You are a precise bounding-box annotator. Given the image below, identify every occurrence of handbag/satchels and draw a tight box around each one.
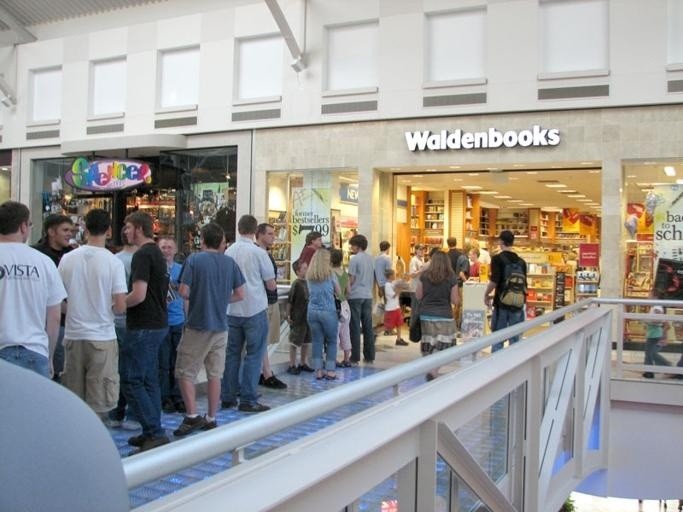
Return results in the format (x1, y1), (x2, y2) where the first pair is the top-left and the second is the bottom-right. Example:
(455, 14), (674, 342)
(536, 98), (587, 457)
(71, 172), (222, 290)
(335, 297), (341, 318)
(409, 310), (421, 342)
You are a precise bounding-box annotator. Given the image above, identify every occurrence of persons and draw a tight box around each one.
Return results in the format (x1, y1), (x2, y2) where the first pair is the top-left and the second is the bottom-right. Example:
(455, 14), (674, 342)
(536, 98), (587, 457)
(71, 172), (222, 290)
(636, 288), (683, 380)
(484, 231), (527, 353)
(638, 500), (683, 511)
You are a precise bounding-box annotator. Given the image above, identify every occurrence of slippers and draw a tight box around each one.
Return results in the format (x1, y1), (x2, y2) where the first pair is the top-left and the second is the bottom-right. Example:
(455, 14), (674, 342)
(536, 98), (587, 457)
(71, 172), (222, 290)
(326, 375), (336, 380)
(316, 374), (325, 380)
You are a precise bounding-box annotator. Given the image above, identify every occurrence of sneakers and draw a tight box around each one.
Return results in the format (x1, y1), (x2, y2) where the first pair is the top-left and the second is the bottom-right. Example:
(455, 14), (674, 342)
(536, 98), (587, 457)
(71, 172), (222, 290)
(238, 402), (270, 412)
(128, 433), (170, 456)
(164, 400), (186, 414)
(173, 414), (217, 436)
(337, 360), (352, 367)
(259, 373), (287, 389)
(221, 400), (238, 409)
(288, 365), (300, 375)
(110, 420), (141, 430)
(298, 364), (315, 372)
(396, 339), (408, 346)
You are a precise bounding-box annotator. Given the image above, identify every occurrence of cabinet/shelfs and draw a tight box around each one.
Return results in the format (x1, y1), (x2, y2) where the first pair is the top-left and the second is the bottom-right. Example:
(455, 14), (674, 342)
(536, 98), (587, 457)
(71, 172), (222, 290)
(409, 190), (601, 326)
(266, 210), (291, 283)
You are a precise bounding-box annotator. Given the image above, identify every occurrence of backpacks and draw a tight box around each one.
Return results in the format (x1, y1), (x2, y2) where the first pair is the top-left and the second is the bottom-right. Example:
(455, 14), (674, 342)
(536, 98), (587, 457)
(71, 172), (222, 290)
(496, 252), (527, 310)
